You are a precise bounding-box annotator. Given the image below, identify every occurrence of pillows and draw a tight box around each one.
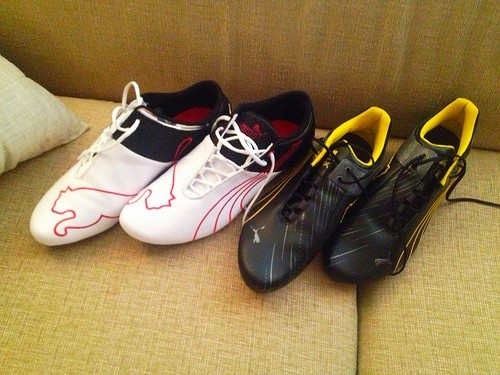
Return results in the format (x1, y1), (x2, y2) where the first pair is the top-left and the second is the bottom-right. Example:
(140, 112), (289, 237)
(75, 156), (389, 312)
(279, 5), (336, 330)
(0, 53), (91, 176)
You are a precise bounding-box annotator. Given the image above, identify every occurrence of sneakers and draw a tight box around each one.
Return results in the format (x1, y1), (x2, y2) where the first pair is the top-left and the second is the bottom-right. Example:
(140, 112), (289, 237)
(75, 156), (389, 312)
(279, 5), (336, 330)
(119, 90), (315, 245)
(322, 98), (479, 284)
(28, 80), (233, 246)
(237, 105), (393, 293)
